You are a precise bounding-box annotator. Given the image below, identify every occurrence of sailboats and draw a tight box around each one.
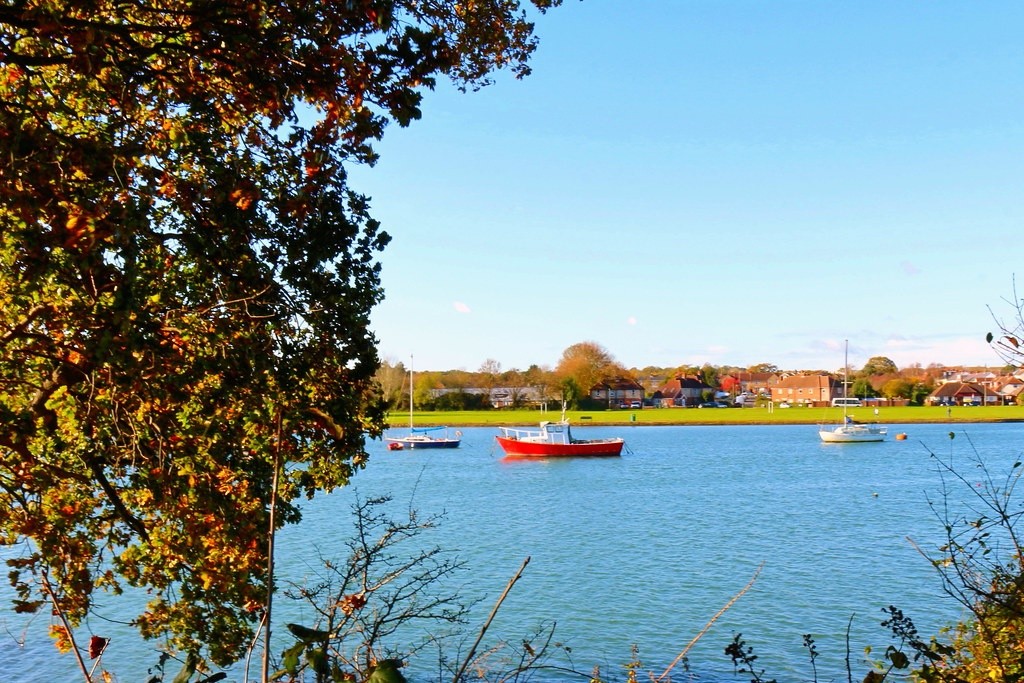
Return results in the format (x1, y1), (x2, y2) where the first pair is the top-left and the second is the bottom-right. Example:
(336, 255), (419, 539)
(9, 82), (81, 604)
(383, 353), (463, 451)
(818, 340), (889, 443)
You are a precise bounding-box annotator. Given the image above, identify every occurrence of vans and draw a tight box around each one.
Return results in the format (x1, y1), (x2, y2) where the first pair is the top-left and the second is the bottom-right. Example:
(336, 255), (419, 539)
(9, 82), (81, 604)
(831, 397), (864, 407)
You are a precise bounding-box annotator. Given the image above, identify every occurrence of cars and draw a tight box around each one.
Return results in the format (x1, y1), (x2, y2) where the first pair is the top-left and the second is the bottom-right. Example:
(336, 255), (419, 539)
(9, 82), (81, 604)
(701, 401), (728, 408)
(620, 401), (630, 409)
(742, 392), (772, 400)
(780, 402), (793, 409)
(631, 400), (641, 408)
(939, 400), (958, 406)
(963, 400), (981, 407)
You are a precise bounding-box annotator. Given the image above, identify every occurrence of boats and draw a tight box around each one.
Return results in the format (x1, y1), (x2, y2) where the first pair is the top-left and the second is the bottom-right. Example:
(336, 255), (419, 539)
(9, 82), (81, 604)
(495, 398), (625, 458)
